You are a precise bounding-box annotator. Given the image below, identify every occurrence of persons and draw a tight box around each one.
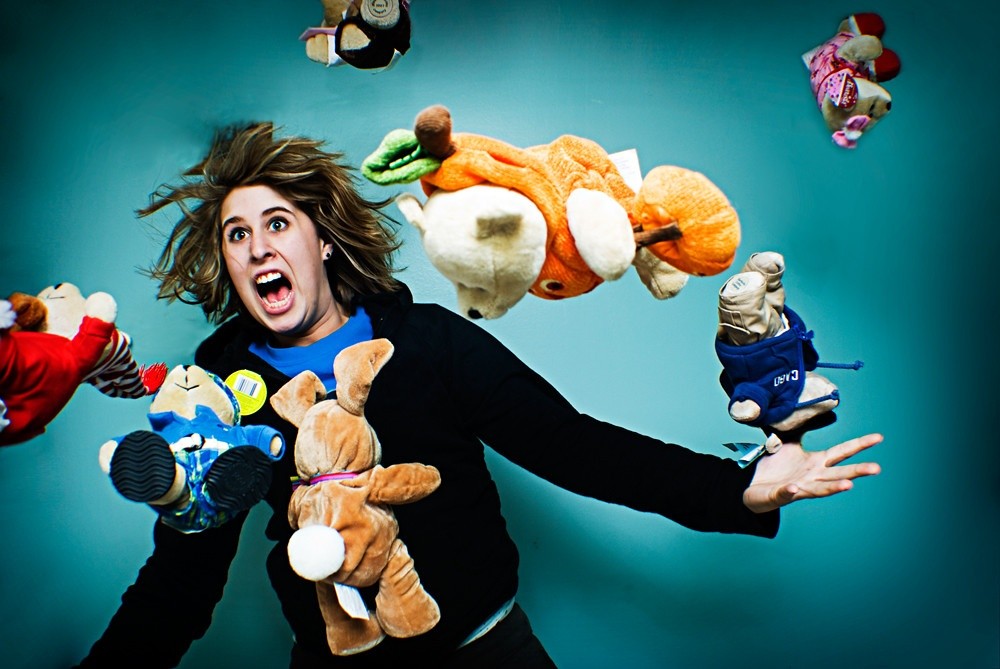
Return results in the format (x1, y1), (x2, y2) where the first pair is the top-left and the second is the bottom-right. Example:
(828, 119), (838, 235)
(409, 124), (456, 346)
(72, 120), (884, 669)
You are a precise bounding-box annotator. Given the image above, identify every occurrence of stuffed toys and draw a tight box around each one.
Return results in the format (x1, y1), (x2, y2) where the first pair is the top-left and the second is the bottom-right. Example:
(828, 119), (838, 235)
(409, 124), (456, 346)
(713, 250), (865, 454)
(359, 104), (742, 321)
(298, 0), (411, 76)
(0, 282), (169, 448)
(269, 338), (441, 655)
(800, 13), (901, 150)
(98, 365), (286, 534)
